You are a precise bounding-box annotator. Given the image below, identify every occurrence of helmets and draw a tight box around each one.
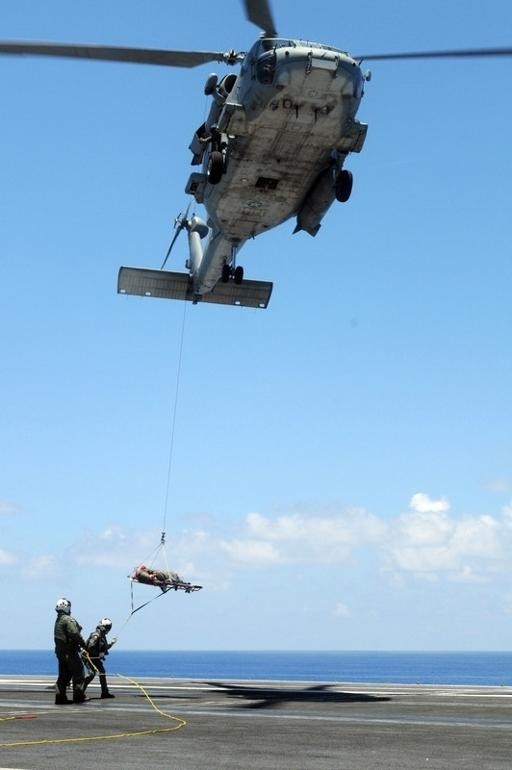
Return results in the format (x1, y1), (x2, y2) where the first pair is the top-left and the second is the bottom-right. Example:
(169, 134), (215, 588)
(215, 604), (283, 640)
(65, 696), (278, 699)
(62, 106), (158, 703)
(55, 598), (71, 615)
(97, 618), (112, 635)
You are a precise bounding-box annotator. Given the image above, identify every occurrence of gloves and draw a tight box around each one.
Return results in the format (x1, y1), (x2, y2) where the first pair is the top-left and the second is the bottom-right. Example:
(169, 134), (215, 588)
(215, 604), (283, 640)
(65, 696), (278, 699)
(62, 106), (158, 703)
(111, 634), (119, 643)
(99, 652), (104, 662)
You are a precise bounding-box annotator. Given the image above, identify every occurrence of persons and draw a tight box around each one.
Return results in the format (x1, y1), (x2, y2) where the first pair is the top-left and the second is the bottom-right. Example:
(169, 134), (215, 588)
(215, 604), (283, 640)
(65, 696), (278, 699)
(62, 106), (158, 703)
(54, 598), (117, 705)
(199, 123), (228, 153)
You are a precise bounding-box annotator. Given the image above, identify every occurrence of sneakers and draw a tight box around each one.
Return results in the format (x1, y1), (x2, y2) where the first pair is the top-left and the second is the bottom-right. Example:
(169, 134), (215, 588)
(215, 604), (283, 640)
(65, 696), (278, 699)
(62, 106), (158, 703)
(101, 693), (115, 699)
(55, 694), (90, 704)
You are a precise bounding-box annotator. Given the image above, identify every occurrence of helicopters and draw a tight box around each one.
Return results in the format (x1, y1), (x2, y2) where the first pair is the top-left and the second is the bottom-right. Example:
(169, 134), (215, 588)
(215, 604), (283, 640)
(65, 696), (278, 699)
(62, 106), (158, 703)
(0, 0), (512, 310)
